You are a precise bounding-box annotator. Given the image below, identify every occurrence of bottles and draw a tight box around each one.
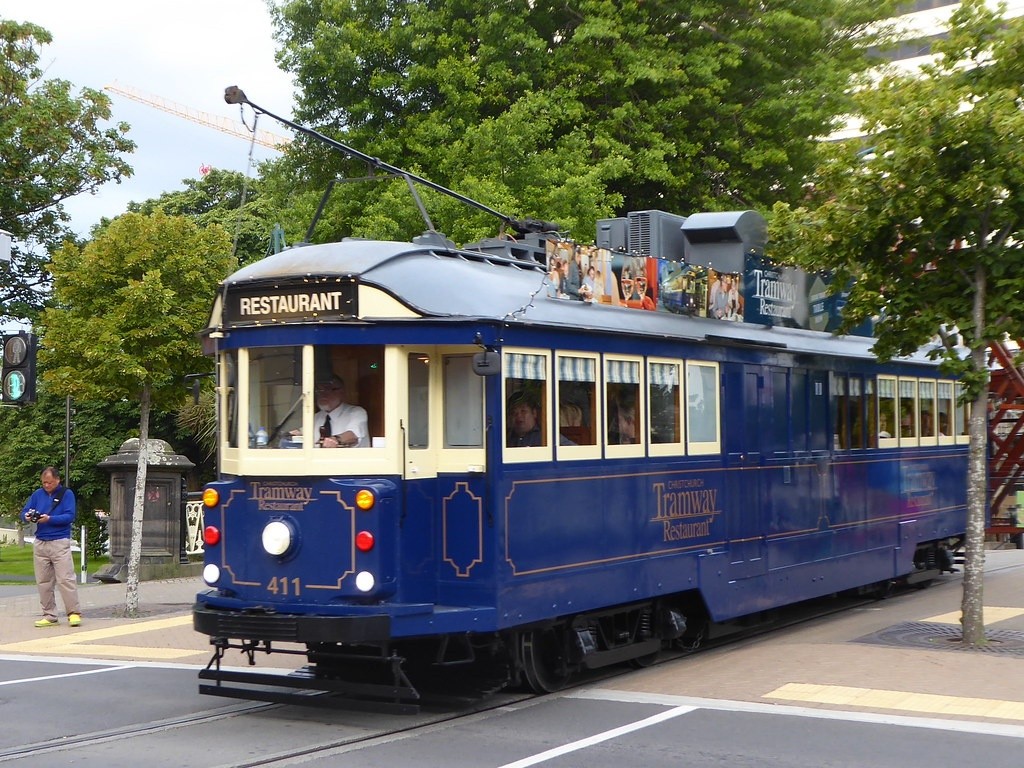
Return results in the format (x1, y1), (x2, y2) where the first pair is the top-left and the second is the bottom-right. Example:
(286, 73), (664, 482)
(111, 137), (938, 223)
(255, 426), (269, 449)
(249, 426), (254, 447)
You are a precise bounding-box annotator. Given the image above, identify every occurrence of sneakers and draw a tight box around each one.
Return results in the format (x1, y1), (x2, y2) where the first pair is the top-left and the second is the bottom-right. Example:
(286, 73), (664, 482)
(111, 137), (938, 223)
(69, 614), (81, 626)
(34, 619), (60, 626)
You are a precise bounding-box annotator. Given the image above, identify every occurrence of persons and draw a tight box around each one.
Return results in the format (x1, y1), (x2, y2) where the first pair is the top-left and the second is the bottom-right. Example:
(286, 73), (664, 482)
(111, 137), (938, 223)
(506, 376), (678, 446)
(281, 372), (373, 450)
(544, 237), (748, 323)
(21, 465), (83, 627)
(877, 397), (952, 440)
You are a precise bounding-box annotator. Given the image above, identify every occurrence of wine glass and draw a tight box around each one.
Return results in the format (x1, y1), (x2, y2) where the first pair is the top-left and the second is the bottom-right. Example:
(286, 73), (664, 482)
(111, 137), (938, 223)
(620, 258), (634, 308)
(633, 258), (649, 309)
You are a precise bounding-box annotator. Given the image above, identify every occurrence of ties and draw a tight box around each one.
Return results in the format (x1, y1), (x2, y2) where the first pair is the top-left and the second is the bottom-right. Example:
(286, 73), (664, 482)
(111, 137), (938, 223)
(324, 415), (331, 437)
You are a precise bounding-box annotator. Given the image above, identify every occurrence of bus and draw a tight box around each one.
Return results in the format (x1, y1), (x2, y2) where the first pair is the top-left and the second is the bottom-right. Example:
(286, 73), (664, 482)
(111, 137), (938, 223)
(187, 231), (969, 710)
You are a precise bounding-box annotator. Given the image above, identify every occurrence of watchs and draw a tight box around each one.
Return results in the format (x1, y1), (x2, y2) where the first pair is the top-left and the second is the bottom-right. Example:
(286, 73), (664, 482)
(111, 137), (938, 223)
(333, 435), (342, 445)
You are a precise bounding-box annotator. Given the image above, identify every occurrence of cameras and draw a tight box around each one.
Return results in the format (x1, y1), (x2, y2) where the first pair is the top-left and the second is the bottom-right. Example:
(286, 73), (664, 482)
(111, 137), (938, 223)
(28, 509), (42, 523)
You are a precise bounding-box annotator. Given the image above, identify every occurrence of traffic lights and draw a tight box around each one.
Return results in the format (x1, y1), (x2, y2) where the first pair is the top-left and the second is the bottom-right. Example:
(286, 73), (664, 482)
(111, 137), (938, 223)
(0, 330), (36, 403)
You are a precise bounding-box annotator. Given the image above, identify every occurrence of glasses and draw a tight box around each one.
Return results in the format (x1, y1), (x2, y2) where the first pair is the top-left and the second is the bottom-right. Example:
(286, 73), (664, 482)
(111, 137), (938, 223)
(315, 387), (341, 393)
